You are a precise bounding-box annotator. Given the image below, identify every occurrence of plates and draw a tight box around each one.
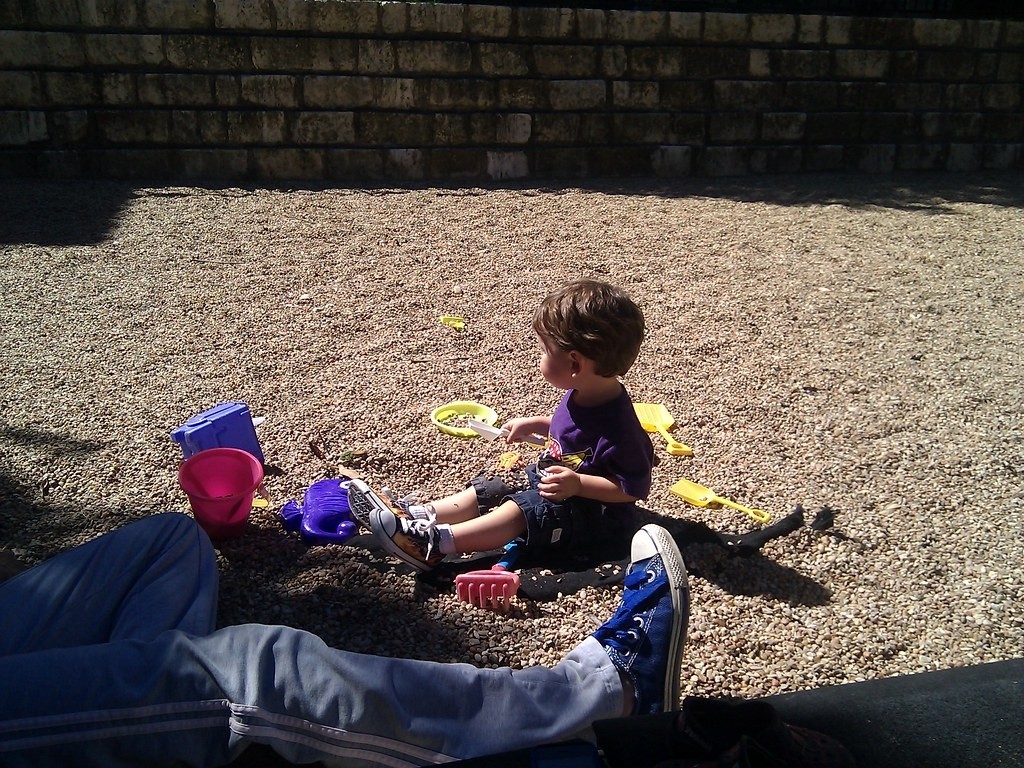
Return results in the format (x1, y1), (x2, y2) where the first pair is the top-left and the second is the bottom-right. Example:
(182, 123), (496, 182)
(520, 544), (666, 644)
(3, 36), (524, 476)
(431, 402), (498, 438)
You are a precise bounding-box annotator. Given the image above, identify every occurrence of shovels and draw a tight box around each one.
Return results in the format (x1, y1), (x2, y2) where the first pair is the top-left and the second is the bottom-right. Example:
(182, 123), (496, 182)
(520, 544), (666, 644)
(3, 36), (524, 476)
(669, 478), (771, 523)
(468, 419), (545, 446)
(632, 402), (693, 456)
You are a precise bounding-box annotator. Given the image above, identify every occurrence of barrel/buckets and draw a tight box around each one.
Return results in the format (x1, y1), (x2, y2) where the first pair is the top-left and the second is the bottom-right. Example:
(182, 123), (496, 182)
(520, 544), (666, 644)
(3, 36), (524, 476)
(178, 448), (264, 534)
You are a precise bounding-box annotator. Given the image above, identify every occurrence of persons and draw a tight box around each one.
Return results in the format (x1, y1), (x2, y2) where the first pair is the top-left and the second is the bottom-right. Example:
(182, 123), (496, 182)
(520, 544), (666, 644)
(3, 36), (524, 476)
(347, 279), (658, 574)
(0, 513), (691, 768)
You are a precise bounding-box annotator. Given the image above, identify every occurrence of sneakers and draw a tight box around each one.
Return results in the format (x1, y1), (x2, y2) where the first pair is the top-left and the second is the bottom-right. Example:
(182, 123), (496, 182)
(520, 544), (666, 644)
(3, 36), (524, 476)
(346, 479), (447, 573)
(589, 522), (691, 721)
